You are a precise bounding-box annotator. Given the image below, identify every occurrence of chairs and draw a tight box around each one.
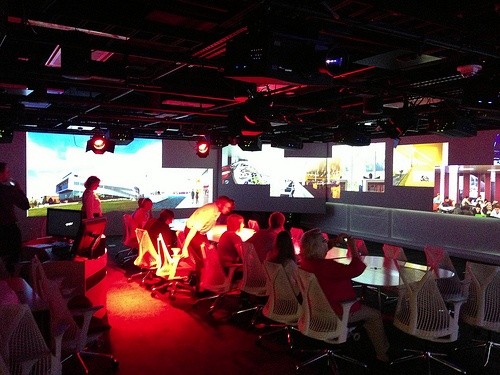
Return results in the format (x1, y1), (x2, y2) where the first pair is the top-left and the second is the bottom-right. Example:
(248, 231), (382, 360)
(388, 264), (467, 375)
(424, 245), (472, 304)
(117, 204), (409, 319)
(255, 261), (303, 354)
(0, 260), (124, 375)
(294, 268), (371, 375)
(451, 261), (500, 369)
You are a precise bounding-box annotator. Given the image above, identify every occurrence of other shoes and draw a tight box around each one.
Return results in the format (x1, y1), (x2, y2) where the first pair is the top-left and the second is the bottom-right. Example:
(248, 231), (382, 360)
(374, 357), (397, 369)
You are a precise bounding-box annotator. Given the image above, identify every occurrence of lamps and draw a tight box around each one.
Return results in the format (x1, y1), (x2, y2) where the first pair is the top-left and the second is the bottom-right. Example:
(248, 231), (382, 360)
(90, 137), (107, 155)
(197, 142), (210, 158)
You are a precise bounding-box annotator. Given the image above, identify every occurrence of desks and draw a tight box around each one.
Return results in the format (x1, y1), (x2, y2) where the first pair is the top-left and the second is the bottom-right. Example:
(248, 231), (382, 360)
(165, 217), (432, 340)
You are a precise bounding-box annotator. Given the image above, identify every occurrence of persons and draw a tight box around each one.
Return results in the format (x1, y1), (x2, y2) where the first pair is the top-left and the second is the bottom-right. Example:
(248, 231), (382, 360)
(461, 195), (500, 219)
(442, 196), (453, 214)
(137, 198), (153, 219)
(218, 214), (251, 301)
(0, 162), (30, 274)
(266, 230), (303, 305)
(182, 196), (235, 300)
(245, 212), (285, 263)
(301, 228), (393, 366)
(142, 209), (174, 267)
(131, 198), (153, 229)
(80, 176), (102, 225)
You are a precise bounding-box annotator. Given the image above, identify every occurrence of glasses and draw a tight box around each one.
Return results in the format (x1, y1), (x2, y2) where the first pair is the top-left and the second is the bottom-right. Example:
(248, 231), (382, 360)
(322, 238), (327, 243)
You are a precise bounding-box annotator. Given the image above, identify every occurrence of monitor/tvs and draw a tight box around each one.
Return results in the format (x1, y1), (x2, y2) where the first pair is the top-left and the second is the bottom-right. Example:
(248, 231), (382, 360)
(46, 208), (82, 240)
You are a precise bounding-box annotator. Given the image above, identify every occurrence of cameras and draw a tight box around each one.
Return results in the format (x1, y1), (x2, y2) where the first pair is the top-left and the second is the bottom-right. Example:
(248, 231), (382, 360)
(343, 237), (348, 244)
(9, 180), (15, 187)
